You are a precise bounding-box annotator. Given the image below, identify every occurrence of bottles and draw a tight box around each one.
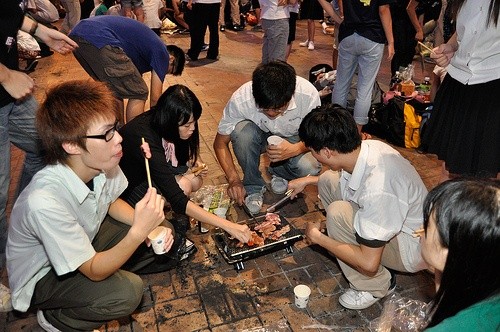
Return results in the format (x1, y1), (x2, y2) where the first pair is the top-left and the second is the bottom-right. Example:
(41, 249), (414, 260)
(420, 77), (432, 93)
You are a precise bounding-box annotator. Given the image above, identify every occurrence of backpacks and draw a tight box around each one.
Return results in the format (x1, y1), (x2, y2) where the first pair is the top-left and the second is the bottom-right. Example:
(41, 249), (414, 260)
(383, 98), (422, 149)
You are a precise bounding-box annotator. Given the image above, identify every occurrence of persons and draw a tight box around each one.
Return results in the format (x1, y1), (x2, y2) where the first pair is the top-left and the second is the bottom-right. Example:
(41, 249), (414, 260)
(213, 58), (321, 214)
(285, 103), (433, 310)
(288, 0), (343, 71)
(118, 84), (253, 244)
(6, 77), (175, 332)
(2, 0), (79, 315)
(412, 178), (500, 332)
(320, 0), (395, 140)
(390, 0), (424, 78)
(16, 0), (261, 131)
(257, 0), (291, 62)
(418, 1), (500, 185)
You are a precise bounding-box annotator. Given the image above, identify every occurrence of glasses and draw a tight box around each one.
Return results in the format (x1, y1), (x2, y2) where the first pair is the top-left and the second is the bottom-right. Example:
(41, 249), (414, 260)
(78, 119), (120, 142)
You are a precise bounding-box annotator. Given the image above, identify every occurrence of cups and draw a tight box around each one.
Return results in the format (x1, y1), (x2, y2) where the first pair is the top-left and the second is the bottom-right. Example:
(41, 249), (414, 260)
(267, 135), (284, 145)
(293, 285), (311, 308)
(148, 225), (168, 255)
(245, 193), (264, 214)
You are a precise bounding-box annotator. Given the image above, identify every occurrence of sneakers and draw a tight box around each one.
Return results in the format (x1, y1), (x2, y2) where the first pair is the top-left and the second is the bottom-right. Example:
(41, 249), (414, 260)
(308, 41), (314, 49)
(244, 185), (268, 214)
(338, 270), (397, 309)
(36, 310), (100, 332)
(271, 174), (288, 195)
(298, 40), (309, 46)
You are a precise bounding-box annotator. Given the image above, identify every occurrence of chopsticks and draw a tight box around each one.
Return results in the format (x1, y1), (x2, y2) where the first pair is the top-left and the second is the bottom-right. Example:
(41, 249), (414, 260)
(284, 173), (311, 196)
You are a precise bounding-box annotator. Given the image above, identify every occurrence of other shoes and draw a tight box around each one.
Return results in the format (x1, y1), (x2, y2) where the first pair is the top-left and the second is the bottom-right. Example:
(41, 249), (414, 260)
(233, 25), (240, 31)
(220, 25), (225, 31)
